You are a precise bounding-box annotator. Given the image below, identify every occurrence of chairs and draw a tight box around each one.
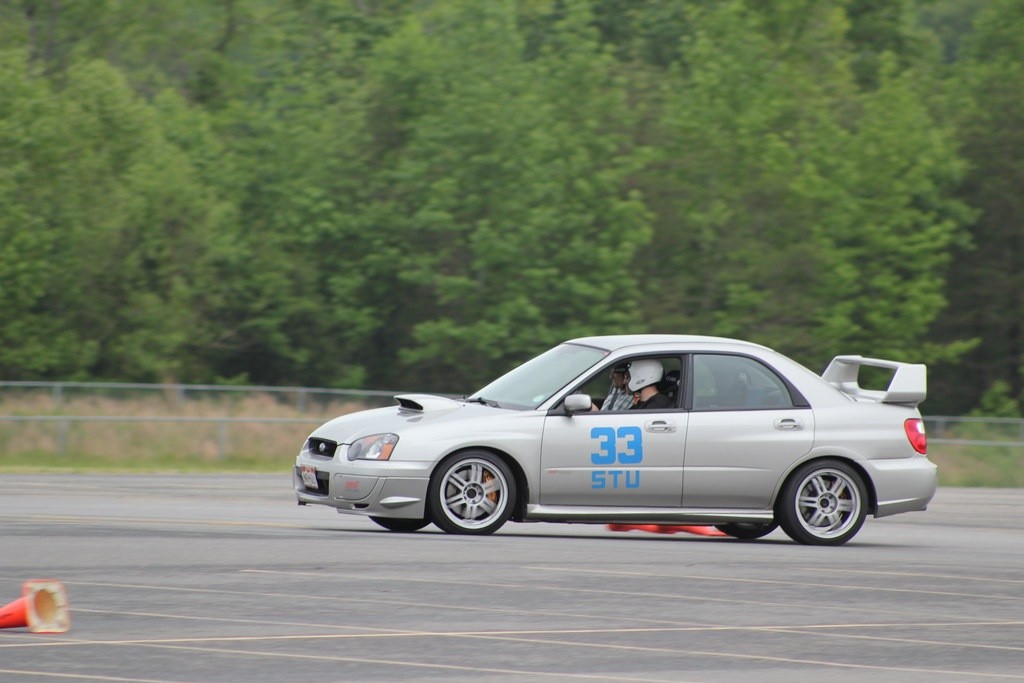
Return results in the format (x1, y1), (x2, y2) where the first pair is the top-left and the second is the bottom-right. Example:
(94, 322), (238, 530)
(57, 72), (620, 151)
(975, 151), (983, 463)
(660, 370), (680, 408)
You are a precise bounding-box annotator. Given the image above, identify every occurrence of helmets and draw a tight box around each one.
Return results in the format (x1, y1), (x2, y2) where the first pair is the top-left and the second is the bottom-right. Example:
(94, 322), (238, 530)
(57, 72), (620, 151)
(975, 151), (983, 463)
(625, 358), (663, 391)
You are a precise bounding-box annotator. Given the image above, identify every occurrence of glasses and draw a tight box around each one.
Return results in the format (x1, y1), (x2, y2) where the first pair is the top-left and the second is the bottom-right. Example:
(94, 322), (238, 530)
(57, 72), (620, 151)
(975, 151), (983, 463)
(611, 367), (626, 372)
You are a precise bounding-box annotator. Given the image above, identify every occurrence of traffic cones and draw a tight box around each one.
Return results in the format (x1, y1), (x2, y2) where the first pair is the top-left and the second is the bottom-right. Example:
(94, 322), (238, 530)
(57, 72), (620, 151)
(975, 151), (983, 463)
(0, 576), (71, 635)
(607, 523), (727, 537)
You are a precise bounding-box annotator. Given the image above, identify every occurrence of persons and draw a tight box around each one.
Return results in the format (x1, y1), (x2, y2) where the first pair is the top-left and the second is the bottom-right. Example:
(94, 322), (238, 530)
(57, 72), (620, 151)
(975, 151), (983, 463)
(568, 359), (673, 411)
(600, 363), (640, 410)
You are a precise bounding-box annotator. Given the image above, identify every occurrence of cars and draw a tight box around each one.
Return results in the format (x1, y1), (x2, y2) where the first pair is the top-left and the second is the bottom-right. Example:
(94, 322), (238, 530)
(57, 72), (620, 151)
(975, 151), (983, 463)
(293, 332), (938, 550)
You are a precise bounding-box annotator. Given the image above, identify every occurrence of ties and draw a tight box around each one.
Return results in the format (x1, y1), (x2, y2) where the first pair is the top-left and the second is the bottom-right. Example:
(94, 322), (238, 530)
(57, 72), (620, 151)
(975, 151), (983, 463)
(608, 392), (619, 411)
